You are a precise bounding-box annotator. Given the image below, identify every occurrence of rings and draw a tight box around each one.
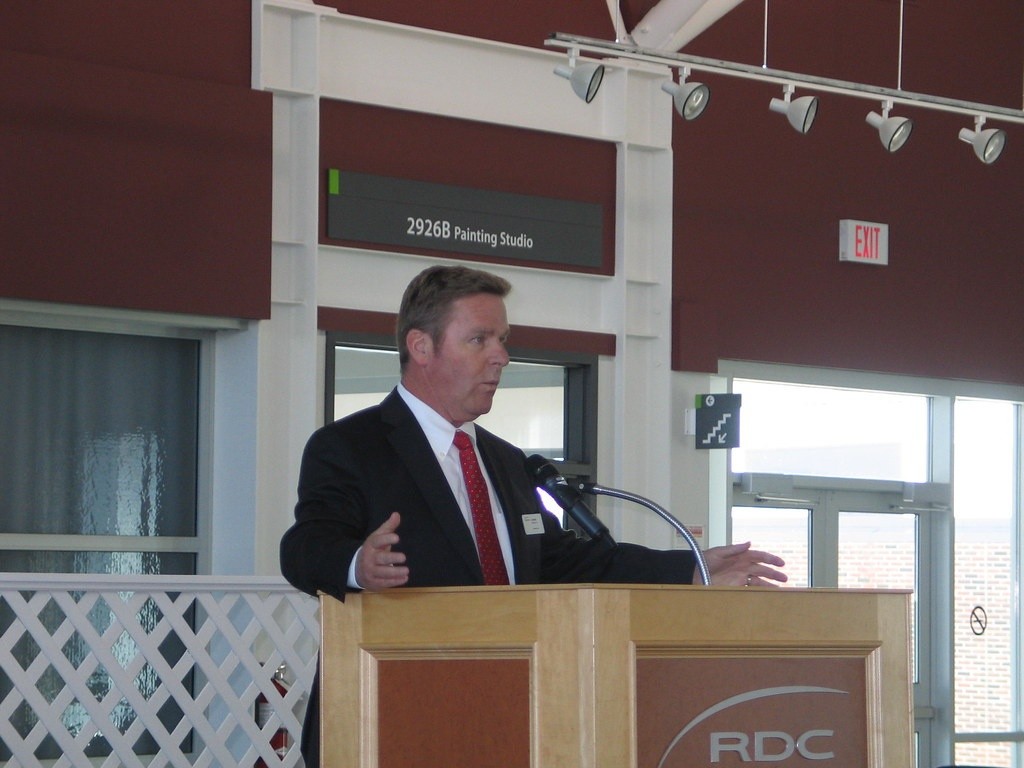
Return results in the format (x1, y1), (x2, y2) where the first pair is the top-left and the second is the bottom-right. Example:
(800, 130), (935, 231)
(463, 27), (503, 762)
(746, 575), (753, 586)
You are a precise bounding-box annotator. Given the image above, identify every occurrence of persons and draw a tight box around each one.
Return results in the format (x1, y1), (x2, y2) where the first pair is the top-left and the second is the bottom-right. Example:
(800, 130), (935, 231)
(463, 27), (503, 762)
(277, 265), (786, 768)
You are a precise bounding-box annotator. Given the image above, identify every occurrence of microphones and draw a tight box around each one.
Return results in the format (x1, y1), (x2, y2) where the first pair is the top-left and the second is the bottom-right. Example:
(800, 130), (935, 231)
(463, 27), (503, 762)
(521, 454), (618, 551)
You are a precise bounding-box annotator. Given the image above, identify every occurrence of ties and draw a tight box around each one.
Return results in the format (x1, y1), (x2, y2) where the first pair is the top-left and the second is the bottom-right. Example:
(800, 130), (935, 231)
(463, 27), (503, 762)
(452, 431), (510, 586)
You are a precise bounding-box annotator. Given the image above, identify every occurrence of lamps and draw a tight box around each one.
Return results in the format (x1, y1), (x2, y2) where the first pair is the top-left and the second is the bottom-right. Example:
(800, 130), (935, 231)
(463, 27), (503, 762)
(768, 94), (820, 135)
(551, 62), (605, 103)
(863, 110), (914, 154)
(661, 79), (710, 122)
